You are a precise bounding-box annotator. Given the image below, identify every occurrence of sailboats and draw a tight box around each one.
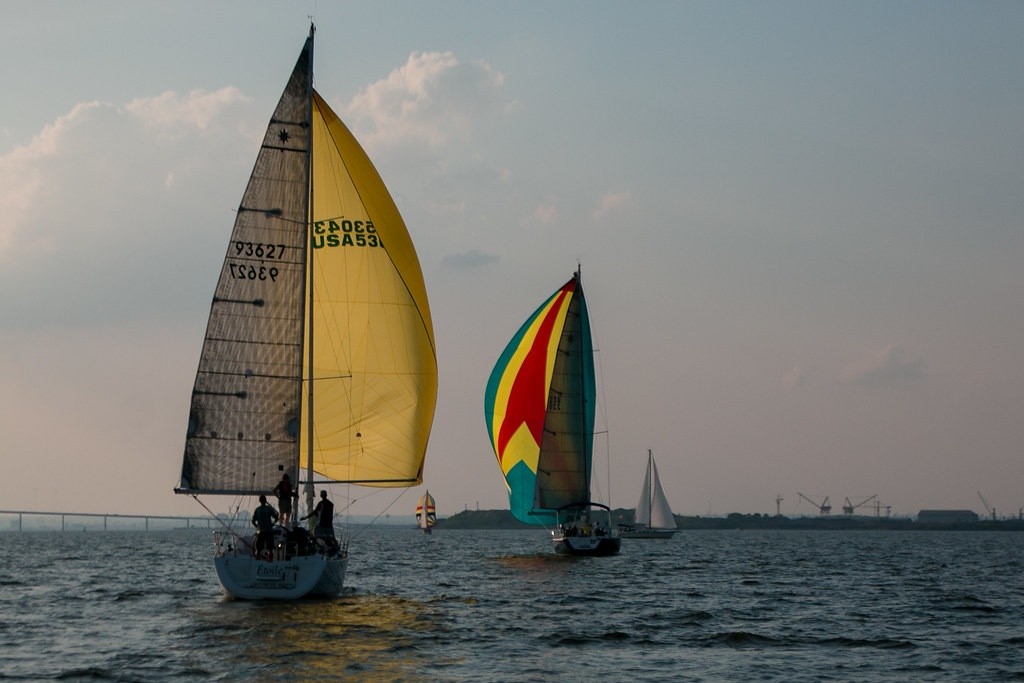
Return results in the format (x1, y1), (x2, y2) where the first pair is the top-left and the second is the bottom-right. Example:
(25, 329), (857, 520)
(484, 263), (622, 557)
(415, 489), (437, 534)
(616, 449), (679, 539)
(173, 20), (439, 602)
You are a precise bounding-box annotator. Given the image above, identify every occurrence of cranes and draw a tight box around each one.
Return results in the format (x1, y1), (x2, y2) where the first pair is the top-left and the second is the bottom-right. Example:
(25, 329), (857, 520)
(796, 491), (832, 514)
(977, 491), (995, 521)
(843, 495), (894, 517)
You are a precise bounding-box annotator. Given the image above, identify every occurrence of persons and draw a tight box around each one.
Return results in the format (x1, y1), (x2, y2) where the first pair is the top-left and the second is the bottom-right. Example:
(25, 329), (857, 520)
(300, 490), (342, 557)
(593, 522), (600, 536)
(273, 474), (299, 527)
(252, 495), (279, 561)
